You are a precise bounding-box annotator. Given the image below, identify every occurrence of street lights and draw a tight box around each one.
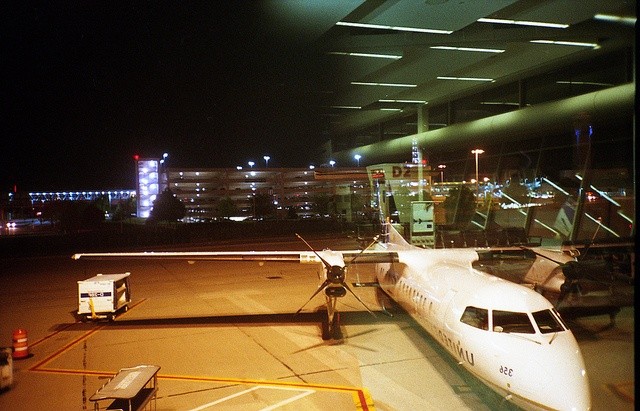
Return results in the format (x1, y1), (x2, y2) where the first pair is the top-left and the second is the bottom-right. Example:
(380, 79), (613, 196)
(247, 160), (255, 168)
(471, 148), (486, 195)
(263, 156), (271, 167)
(236, 166), (243, 170)
(329, 160), (336, 167)
(437, 165), (447, 184)
(354, 154), (362, 166)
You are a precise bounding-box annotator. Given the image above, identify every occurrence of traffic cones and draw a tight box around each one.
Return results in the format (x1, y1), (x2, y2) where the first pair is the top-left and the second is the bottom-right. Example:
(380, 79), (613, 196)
(11, 327), (32, 362)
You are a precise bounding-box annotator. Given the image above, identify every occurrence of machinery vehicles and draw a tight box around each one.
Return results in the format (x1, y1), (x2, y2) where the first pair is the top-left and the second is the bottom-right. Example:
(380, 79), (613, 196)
(76, 272), (133, 322)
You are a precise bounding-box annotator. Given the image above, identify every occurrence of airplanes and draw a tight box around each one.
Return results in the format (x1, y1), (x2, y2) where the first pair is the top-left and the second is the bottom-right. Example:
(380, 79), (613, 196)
(70, 162), (636, 411)
